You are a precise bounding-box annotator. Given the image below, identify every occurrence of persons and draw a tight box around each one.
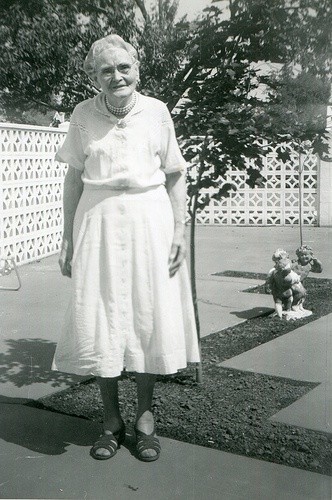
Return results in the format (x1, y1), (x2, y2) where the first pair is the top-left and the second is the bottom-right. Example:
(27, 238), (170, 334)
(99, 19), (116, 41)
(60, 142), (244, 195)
(52, 33), (202, 462)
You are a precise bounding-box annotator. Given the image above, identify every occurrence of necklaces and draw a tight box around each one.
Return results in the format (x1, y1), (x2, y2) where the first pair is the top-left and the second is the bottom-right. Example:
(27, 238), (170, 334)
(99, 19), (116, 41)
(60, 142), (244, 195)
(105, 93), (136, 116)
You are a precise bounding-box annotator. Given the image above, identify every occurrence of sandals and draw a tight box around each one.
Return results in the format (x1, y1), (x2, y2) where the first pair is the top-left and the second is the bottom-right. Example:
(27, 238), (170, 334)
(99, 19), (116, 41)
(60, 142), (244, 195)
(90, 419), (127, 461)
(134, 423), (161, 461)
